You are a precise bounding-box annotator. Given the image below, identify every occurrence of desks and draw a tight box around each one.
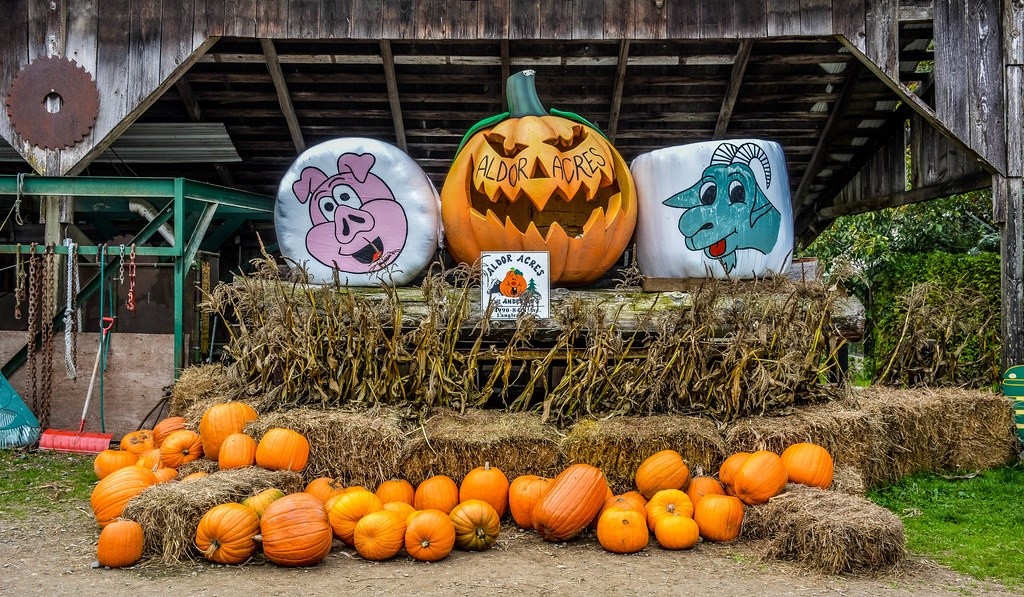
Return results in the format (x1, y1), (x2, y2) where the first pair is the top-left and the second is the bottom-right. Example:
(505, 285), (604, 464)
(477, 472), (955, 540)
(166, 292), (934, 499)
(235, 277), (865, 381)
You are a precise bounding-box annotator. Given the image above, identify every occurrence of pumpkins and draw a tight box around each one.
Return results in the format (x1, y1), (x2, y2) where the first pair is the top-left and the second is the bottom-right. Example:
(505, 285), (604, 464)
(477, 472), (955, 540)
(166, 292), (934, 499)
(90, 403), (308, 566)
(195, 442), (834, 566)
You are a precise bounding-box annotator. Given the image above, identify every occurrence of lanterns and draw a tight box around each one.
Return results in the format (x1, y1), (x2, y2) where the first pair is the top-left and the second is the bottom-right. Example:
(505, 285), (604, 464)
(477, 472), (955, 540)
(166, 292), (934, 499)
(441, 69), (637, 280)
